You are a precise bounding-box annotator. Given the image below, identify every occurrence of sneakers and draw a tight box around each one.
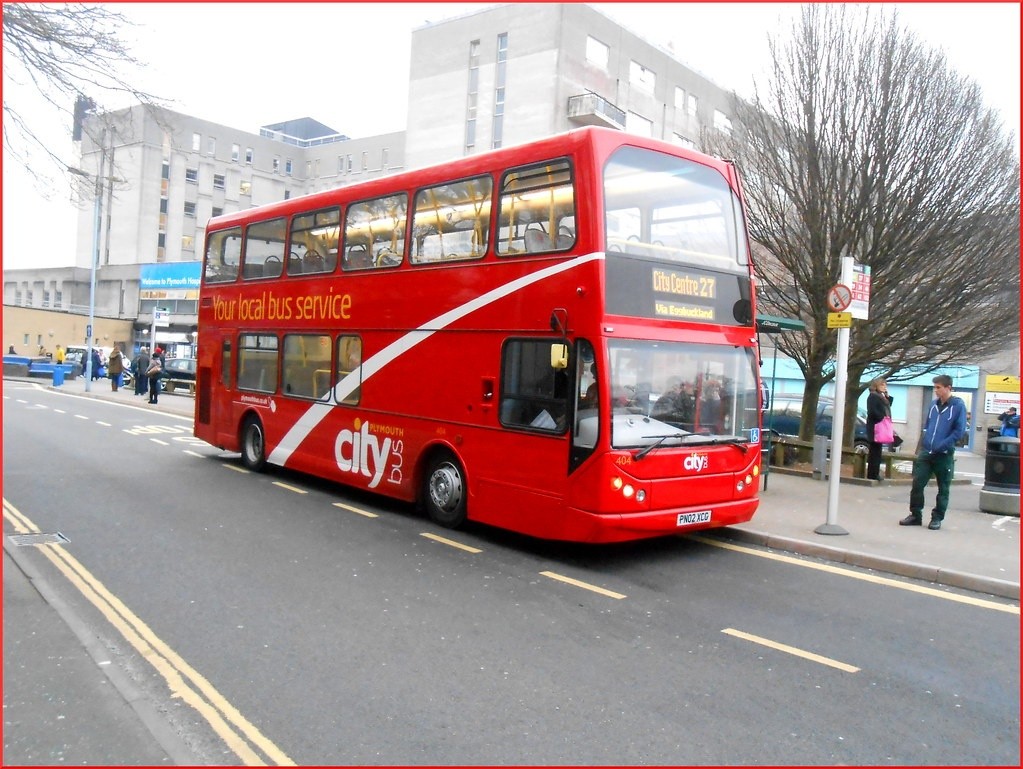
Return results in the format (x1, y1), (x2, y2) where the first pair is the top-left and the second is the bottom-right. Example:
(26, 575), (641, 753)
(929, 517), (942, 530)
(900, 515), (922, 525)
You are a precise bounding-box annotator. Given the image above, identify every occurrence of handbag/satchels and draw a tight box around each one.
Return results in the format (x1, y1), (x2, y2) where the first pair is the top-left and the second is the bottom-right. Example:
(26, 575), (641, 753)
(147, 361), (162, 377)
(98, 367), (105, 376)
(118, 374), (124, 388)
(874, 415), (895, 443)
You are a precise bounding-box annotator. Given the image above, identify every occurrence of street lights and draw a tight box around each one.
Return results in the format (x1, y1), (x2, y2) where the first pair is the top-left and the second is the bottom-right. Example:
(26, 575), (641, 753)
(67, 167), (126, 393)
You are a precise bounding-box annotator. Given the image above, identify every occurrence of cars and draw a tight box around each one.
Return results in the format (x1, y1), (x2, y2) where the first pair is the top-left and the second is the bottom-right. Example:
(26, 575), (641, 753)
(157, 357), (198, 396)
(714, 390), (905, 464)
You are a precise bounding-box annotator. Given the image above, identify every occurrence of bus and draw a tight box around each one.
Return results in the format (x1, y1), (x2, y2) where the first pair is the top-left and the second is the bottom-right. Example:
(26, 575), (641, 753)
(190, 123), (766, 559)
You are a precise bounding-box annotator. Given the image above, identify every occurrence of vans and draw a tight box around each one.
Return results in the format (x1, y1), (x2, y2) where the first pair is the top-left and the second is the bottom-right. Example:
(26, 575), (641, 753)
(64, 344), (135, 386)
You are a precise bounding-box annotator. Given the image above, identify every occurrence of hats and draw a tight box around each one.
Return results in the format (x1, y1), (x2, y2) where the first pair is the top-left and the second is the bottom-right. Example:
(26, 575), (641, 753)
(156, 348), (162, 353)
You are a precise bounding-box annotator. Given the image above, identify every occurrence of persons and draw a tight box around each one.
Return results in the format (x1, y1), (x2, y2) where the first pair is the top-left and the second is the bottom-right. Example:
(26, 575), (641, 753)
(530, 360), (624, 426)
(80, 349), (105, 381)
(900, 375), (967, 530)
(39, 345), (46, 356)
(109, 347), (123, 391)
(967, 412), (970, 428)
(650, 377), (728, 434)
(55, 345), (64, 364)
(998, 408), (1020, 437)
(867, 378), (893, 480)
(131, 346), (170, 403)
(9, 345), (16, 354)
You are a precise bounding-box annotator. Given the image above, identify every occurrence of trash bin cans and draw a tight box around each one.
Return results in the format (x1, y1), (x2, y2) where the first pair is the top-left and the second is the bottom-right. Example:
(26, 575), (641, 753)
(982, 436), (1021, 494)
(987, 426), (1001, 438)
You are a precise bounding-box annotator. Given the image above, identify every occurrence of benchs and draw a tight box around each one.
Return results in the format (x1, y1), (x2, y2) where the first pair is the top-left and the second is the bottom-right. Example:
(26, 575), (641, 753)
(225, 356), (343, 398)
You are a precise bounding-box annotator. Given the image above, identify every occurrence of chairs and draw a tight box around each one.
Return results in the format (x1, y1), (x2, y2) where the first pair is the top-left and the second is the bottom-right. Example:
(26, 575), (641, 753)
(523, 218), (665, 254)
(263, 244), (400, 276)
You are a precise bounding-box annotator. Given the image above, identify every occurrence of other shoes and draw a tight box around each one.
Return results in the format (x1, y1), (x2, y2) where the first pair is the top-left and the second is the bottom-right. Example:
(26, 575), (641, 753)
(867, 474), (884, 481)
(149, 401), (158, 404)
